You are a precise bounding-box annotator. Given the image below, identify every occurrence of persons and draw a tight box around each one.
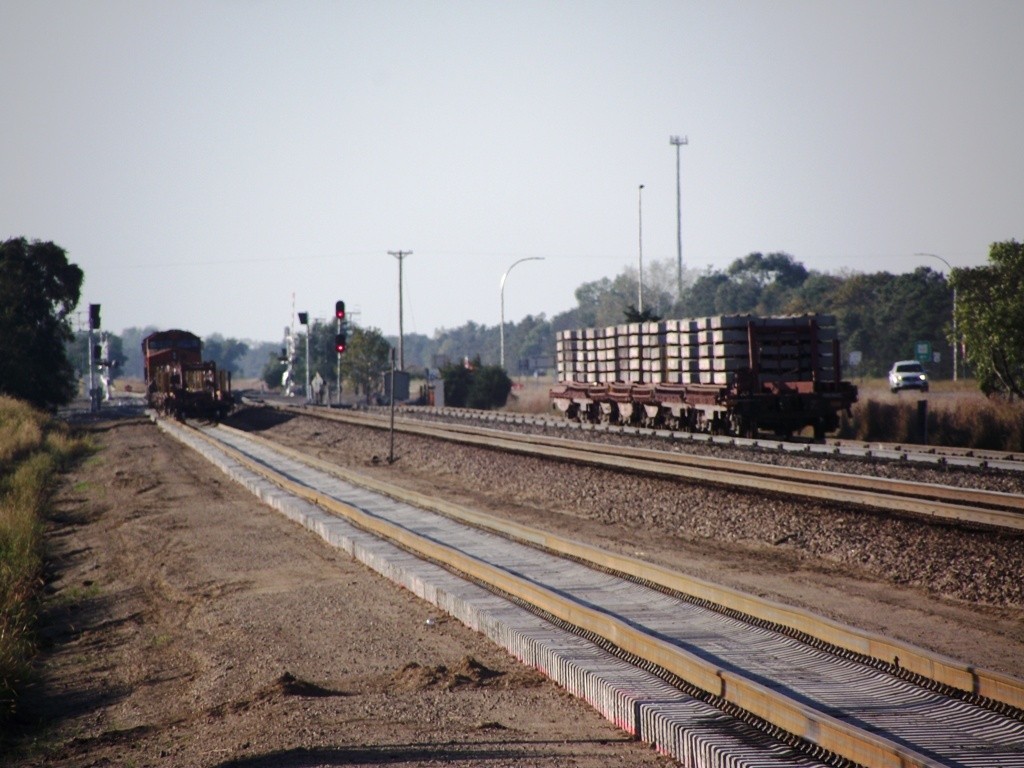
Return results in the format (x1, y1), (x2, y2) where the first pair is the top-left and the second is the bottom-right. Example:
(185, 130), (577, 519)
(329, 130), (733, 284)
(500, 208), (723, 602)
(169, 385), (188, 423)
(312, 372), (325, 404)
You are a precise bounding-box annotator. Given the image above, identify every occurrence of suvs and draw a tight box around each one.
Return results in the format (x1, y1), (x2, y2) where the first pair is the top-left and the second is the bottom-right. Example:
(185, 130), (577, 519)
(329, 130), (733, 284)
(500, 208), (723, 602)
(888, 359), (932, 395)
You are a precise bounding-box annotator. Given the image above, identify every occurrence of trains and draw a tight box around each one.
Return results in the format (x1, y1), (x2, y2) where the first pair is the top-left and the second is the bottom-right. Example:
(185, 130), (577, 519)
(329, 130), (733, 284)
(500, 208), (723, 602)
(546, 313), (859, 445)
(139, 328), (228, 427)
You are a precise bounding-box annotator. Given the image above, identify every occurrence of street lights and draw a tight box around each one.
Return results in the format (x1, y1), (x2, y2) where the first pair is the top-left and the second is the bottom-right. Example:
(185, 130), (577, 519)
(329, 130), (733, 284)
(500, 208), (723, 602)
(635, 182), (646, 319)
(914, 251), (957, 381)
(385, 249), (414, 370)
(499, 255), (548, 369)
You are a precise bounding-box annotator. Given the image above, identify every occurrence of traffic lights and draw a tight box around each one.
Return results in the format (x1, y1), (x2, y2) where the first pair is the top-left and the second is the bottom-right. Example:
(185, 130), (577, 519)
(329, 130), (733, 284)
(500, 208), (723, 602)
(91, 303), (101, 329)
(336, 300), (345, 320)
(95, 344), (102, 360)
(336, 333), (345, 353)
(297, 312), (308, 325)
